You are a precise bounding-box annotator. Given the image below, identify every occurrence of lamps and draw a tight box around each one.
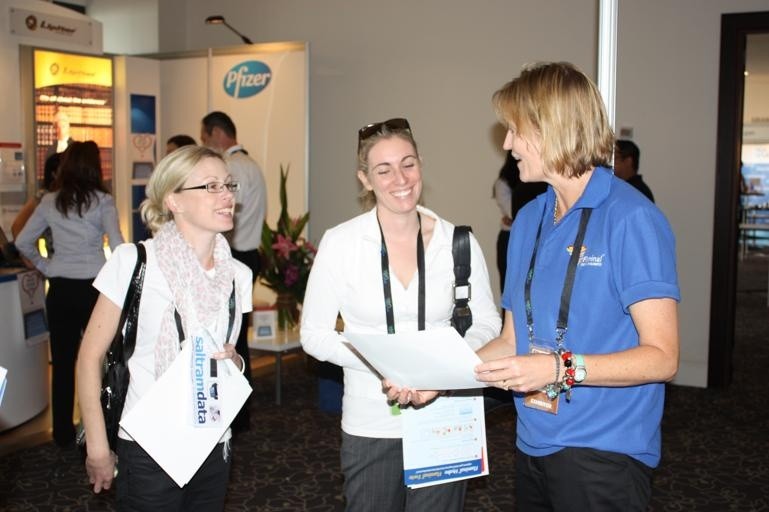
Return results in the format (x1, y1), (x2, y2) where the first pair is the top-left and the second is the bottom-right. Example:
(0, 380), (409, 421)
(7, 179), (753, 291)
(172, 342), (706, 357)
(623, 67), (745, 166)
(204, 15), (251, 46)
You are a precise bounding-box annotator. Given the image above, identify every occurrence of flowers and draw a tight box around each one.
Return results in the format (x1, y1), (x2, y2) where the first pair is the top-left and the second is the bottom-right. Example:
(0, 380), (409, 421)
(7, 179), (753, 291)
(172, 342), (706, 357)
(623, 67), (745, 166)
(256, 161), (317, 305)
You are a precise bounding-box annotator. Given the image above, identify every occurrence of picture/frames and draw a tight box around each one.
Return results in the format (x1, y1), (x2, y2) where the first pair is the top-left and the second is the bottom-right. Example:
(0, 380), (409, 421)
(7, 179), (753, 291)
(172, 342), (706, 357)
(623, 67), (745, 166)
(34, 46), (116, 198)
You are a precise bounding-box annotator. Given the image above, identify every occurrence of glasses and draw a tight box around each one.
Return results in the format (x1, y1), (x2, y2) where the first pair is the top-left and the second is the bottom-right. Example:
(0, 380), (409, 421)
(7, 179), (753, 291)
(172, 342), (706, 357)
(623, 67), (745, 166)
(358, 118), (414, 153)
(182, 181), (237, 193)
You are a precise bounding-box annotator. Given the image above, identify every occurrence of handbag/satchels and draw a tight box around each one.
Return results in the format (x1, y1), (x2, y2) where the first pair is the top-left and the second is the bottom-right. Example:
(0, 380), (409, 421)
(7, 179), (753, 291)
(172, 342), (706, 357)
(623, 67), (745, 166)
(74, 349), (128, 445)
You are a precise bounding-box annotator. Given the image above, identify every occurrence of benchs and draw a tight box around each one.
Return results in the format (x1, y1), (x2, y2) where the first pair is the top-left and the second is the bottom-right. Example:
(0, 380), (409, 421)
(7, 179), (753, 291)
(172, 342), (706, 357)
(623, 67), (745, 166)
(739, 190), (769, 260)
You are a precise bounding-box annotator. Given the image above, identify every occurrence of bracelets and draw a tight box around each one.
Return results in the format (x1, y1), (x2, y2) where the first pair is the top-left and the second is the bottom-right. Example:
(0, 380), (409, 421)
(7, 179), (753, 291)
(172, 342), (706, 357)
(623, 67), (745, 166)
(560, 351), (575, 391)
(551, 351), (560, 385)
(237, 354), (245, 373)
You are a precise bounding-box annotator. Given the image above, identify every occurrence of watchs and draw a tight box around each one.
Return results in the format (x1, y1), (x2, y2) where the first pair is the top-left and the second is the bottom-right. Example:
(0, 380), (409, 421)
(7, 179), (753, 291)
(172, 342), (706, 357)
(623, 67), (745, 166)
(573, 354), (587, 383)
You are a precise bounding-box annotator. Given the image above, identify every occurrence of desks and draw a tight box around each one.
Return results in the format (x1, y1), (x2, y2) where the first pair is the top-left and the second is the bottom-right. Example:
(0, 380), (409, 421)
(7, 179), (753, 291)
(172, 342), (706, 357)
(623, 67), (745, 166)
(248, 339), (301, 406)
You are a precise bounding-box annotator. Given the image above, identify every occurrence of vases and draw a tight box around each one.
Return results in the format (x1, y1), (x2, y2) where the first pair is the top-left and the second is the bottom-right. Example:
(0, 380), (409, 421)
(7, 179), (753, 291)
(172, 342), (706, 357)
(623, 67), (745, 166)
(274, 303), (300, 339)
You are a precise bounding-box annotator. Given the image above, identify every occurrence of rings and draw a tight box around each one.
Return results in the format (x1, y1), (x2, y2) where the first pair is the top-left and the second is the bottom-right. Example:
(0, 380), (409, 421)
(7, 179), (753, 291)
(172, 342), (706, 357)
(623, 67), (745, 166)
(502, 379), (509, 390)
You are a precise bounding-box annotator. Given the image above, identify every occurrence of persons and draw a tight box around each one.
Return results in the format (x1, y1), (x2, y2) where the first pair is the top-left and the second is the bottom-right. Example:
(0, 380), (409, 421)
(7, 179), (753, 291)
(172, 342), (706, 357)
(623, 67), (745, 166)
(615, 140), (654, 204)
(493, 150), (548, 328)
(383, 60), (681, 512)
(298, 117), (502, 512)
(74, 144), (254, 512)
(166, 134), (196, 154)
(11, 152), (65, 268)
(200, 111), (267, 434)
(13, 140), (125, 450)
